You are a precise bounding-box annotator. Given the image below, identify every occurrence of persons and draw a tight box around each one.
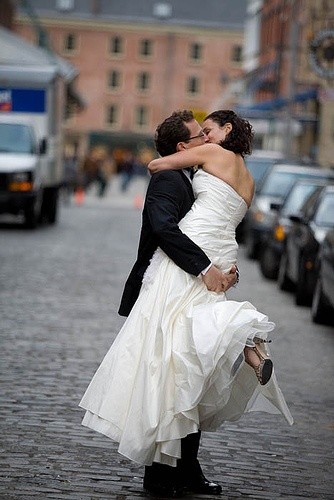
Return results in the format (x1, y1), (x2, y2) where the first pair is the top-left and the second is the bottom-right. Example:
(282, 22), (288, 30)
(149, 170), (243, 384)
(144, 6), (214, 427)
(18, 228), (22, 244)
(84, 145), (135, 197)
(77, 109), (294, 467)
(118, 110), (239, 495)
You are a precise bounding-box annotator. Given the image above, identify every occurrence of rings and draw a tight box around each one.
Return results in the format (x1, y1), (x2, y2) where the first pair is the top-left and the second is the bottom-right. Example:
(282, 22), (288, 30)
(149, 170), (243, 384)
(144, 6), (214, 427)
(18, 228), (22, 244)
(230, 269), (236, 273)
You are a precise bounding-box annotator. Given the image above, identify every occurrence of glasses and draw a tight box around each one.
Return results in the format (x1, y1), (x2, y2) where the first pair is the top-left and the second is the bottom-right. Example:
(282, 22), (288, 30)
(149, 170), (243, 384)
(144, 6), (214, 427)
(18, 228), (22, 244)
(182, 130), (204, 143)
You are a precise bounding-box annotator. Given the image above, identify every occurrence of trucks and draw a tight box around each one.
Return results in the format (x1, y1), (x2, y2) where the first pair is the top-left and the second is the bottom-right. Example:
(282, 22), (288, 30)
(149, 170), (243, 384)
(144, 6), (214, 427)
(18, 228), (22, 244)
(0, 67), (66, 227)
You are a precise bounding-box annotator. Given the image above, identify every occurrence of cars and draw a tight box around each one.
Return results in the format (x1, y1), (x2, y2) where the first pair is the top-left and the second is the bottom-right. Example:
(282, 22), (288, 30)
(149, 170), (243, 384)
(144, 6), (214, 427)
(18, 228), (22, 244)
(278, 177), (334, 326)
(235, 150), (286, 245)
(260, 179), (327, 281)
(245, 164), (334, 259)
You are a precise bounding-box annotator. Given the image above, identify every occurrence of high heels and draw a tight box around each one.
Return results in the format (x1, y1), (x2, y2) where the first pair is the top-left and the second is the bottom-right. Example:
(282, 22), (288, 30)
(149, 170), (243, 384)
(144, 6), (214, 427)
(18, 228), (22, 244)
(243, 336), (273, 386)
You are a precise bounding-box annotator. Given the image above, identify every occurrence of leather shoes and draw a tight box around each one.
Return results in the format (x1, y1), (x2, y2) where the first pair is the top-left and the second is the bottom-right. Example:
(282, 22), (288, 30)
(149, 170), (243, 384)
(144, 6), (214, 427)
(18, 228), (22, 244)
(141, 459), (223, 500)
